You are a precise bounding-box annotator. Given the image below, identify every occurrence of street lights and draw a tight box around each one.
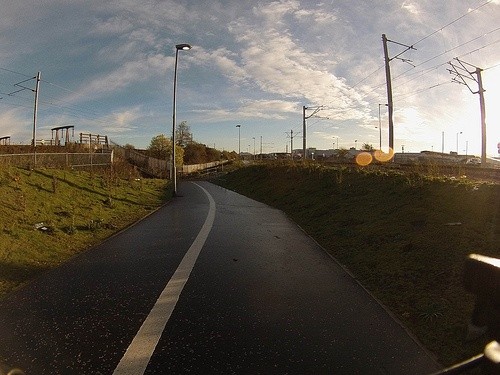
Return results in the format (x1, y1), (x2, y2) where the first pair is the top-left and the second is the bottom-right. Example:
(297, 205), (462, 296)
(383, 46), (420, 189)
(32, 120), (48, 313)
(377, 103), (389, 151)
(171, 43), (192, 196)
(236, 124), (242, 159)
